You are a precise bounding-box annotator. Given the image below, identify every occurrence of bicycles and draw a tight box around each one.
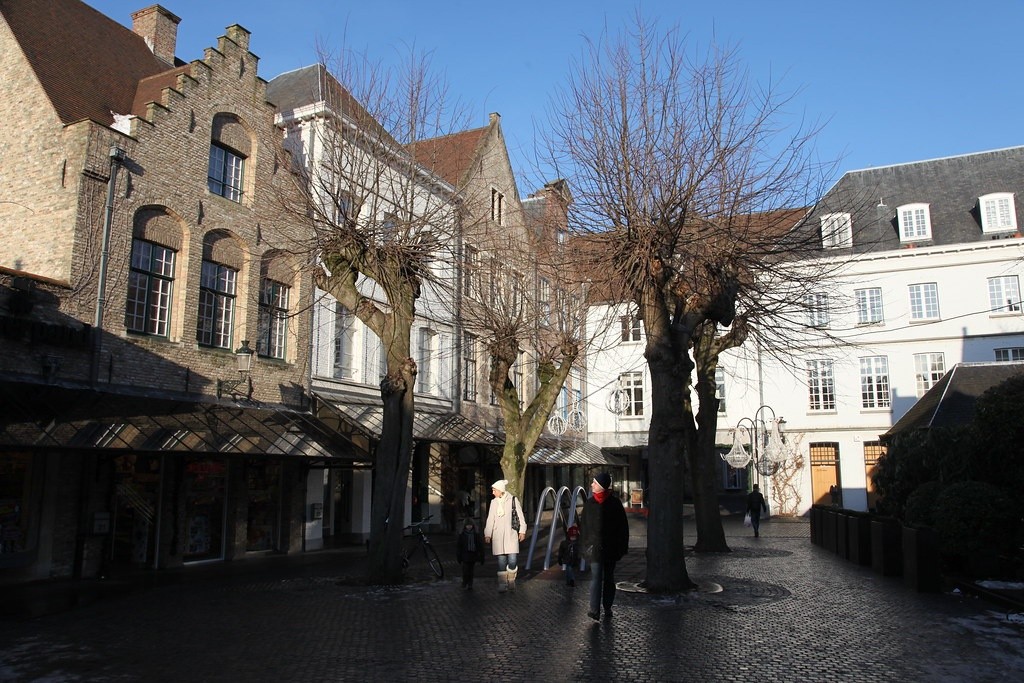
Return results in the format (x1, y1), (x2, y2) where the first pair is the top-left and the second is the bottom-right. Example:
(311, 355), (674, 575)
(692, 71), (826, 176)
(365, 514), (443, 579)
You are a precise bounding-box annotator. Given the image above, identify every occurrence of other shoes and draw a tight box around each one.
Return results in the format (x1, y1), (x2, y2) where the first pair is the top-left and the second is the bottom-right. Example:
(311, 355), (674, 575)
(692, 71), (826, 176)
(605, 608), (613, 616)
(461, 583), (467, 588)
(587, 610), (600, 620)
(468, 585), (472, 589)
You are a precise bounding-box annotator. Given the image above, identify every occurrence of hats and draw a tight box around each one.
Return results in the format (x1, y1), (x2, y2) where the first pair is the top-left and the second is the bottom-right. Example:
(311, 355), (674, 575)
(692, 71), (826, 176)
(594, 473), (611, 490)
(567, 526), (579, 540)
(464, 518), (474, 526)
(492, 480), (508, 492)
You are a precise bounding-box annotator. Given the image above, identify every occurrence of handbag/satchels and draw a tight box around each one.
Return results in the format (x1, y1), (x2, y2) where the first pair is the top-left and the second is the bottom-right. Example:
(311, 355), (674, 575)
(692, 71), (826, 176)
(743, 513), (751, 528)
(511, 496), (520, 530)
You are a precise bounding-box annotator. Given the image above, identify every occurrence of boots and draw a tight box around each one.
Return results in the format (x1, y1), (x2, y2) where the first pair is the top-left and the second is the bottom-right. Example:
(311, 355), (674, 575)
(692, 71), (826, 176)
(570, 579), (574, 587)
(566, 580), (570, 585)
(497, 571), (508, 592)
(506, 564), (518, 590)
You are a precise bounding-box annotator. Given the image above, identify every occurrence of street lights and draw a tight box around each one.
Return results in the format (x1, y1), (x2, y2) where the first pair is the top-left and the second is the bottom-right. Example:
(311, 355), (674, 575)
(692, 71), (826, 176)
(720, 405), (790, 504)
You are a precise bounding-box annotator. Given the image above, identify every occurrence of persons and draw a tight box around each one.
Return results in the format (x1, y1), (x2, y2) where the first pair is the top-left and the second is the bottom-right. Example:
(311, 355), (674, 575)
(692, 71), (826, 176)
(442, 484), (484, 521)
(830, 484), (840, 507)
(581, 473), (631, 622)
(746, 484), (766, 537)
(484, 479), (528, 594)
(556, 525), (582, 587)
(455, 518), (486, 591)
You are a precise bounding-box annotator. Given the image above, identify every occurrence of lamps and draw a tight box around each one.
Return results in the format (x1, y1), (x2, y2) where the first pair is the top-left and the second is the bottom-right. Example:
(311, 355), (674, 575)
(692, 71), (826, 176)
(724, 405), (790, 476)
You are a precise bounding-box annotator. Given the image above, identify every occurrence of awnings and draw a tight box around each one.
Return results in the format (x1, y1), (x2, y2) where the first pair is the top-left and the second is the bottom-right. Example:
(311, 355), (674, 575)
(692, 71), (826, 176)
(1, 392), (628, 480)
